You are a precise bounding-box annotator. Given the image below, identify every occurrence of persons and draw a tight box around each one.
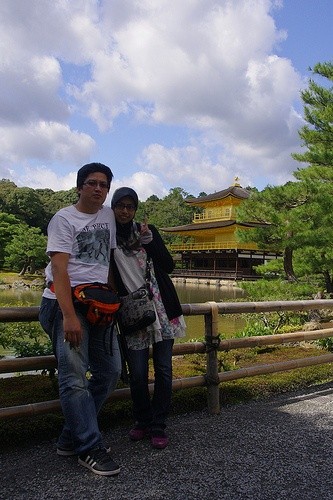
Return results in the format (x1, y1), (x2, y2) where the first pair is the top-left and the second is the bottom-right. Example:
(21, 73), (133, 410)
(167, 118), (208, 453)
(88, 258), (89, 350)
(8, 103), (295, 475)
(39, 163), (120, 476)
(106, 186), (184, 452)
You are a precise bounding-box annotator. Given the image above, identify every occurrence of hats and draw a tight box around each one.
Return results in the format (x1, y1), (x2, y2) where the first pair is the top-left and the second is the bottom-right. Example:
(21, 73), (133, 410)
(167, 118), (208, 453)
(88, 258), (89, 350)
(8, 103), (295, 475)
(111, 187), (138, 209)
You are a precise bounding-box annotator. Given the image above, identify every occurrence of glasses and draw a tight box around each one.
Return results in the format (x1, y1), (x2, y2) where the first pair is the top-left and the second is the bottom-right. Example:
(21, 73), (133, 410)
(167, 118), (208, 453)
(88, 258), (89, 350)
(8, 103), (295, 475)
(117, 204), (136, 210)
(83, 180), (109, 188)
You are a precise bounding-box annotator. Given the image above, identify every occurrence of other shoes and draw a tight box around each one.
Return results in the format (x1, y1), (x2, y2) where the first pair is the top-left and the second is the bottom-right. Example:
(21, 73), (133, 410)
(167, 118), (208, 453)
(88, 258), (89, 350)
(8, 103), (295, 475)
(151, 428), (169, 448)
(131, 426), (151, 441)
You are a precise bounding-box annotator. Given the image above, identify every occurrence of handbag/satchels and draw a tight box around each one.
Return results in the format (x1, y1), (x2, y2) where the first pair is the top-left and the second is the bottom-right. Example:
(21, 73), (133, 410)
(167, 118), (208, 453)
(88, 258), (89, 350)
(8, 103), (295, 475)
(72, 282), (156, 356)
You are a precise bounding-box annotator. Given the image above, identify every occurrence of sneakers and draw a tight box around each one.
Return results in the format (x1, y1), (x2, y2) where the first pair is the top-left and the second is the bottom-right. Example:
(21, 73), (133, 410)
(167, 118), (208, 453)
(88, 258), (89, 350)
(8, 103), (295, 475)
(57, 447), (77, 455)
(78, 446), (120, 475)
